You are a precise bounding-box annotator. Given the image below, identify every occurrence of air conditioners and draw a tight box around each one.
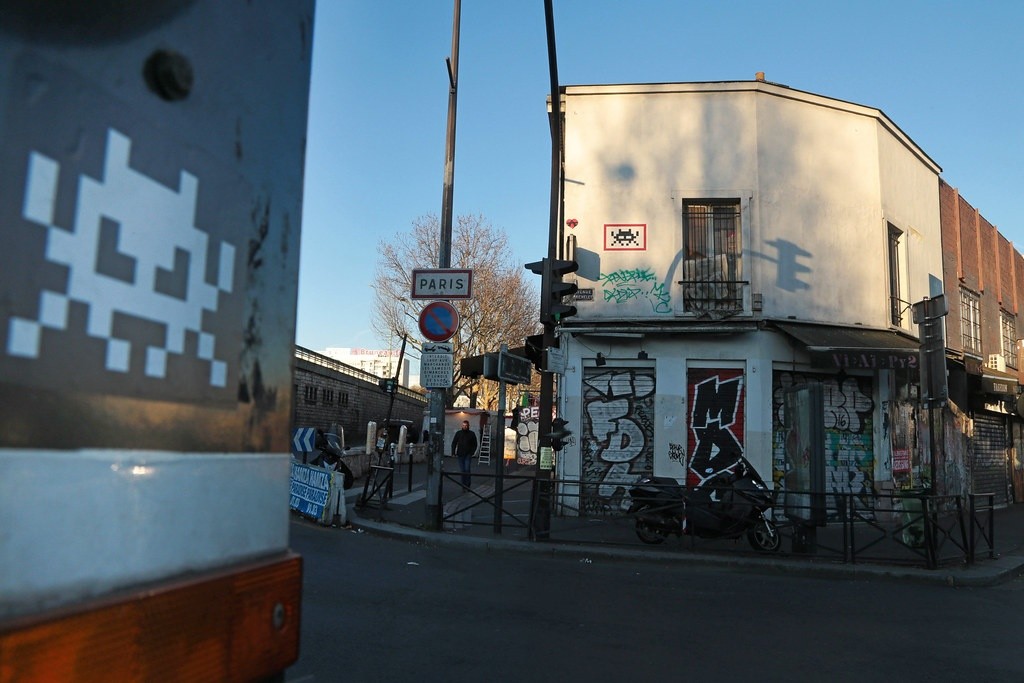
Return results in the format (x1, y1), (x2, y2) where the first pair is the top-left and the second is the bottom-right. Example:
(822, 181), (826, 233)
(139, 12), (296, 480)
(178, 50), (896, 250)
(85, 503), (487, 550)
(989, 354), (1006, 372)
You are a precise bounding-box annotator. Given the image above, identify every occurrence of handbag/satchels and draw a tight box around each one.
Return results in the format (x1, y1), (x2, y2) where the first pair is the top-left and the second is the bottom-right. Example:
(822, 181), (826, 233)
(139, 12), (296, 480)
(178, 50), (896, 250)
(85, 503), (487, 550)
(333, 457), (354, 490)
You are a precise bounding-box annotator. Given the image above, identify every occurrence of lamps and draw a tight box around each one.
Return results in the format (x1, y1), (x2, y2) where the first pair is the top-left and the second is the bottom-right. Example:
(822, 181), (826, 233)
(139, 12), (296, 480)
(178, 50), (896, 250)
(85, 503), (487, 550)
(596, 352), (606, 367)
(638, 351), (648, 359)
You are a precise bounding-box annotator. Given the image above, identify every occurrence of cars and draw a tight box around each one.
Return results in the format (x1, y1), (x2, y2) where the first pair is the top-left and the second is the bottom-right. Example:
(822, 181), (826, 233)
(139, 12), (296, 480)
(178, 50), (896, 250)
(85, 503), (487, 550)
(379, 419), (419, 444)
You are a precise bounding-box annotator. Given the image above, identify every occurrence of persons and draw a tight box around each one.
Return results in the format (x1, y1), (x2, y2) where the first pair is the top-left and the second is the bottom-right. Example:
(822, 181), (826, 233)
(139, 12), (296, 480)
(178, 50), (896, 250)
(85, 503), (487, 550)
(451, 421), (477, 492)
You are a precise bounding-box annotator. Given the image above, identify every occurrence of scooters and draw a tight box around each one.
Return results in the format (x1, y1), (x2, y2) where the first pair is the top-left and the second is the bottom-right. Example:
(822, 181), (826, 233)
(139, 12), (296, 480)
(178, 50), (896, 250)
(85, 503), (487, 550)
(306, 426), (354, 491)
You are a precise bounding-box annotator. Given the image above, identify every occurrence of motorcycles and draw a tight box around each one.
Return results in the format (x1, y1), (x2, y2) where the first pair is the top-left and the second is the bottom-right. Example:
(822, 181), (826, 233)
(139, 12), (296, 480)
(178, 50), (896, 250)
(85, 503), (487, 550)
(624, 452), (782, 553)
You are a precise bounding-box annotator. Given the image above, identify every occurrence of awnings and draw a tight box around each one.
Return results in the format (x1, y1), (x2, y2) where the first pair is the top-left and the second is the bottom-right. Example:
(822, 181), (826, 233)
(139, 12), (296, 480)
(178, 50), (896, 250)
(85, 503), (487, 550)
(461, 347), (526, 386)
(945, 354), (1018, 394)
(772, 321), (920, 353)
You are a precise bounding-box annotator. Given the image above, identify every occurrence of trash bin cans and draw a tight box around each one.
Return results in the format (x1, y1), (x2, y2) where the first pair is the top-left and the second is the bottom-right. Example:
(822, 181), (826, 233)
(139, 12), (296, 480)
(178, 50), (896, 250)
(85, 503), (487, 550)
(899, 488), (931, 548)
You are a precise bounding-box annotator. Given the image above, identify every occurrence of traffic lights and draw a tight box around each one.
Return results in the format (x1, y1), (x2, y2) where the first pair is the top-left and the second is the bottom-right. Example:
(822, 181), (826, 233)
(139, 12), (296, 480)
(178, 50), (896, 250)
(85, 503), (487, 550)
(525, 334), (544, 369)
(524, 257), (548, 324)
(552, 416), (573, 451)
(546, 255), (579, 327)
